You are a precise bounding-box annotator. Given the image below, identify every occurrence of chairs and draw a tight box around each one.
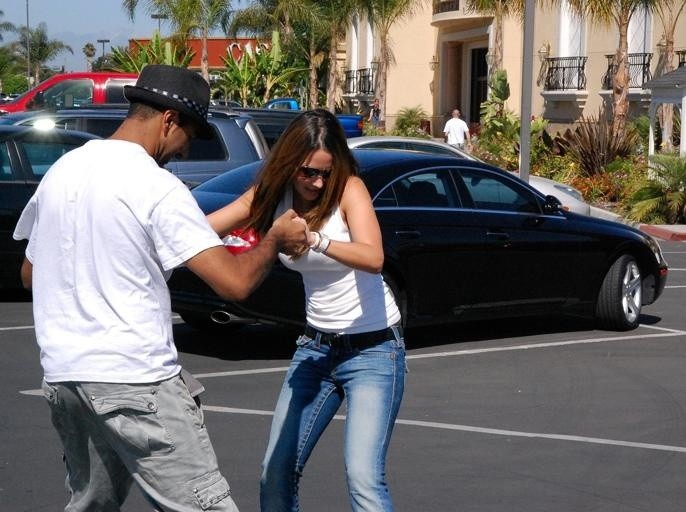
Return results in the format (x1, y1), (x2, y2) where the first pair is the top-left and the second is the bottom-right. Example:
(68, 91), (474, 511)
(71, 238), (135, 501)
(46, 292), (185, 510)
(406, 180), (438, 206)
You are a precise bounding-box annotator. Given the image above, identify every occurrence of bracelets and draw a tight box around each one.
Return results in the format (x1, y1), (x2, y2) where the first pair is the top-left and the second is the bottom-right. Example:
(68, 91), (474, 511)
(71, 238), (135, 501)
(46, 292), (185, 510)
(317, 235), (330, 253)
(309, 231), (321, 250)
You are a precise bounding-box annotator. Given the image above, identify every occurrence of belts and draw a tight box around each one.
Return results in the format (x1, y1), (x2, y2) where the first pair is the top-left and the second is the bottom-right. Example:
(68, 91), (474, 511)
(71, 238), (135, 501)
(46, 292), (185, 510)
(303, 324), (405, 347)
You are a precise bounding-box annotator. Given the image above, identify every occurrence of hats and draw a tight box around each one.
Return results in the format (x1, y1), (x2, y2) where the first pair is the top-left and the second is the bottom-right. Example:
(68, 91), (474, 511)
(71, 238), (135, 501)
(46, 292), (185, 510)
(124, 64), (214, 140)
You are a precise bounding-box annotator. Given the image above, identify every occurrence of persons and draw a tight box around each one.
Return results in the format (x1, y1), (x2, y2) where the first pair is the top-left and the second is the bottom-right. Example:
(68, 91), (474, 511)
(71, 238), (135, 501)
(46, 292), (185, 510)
(203, 109), (410, 512)
(367, 99), (381, 128)
(443, 109), (472, 152)
(11, 65), (308, 512)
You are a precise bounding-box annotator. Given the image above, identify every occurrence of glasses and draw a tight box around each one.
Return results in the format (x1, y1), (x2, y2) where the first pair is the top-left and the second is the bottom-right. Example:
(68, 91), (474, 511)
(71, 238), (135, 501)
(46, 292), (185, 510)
(296, 166), (332, 179)
